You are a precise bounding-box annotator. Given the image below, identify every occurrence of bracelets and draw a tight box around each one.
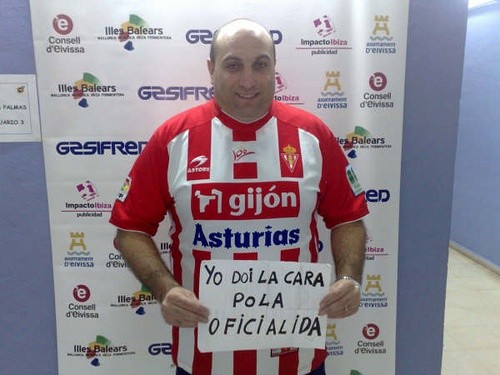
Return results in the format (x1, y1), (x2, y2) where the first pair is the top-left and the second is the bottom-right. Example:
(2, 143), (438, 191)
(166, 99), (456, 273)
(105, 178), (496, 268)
(338, 276), (364, 299)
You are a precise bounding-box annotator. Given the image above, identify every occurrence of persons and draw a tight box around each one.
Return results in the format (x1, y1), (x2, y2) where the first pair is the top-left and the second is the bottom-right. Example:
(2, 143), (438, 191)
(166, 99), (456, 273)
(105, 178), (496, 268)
(105, 19), (369, 375)
